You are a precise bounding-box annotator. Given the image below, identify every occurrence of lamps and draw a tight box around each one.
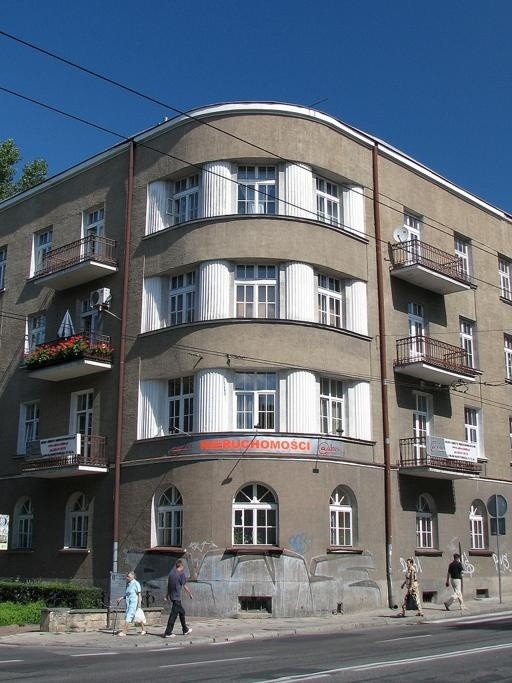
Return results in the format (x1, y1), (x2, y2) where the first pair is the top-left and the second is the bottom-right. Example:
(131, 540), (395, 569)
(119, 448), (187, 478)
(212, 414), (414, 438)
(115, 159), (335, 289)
(254, 423), (262, 432)
(325, 429), (345, 435)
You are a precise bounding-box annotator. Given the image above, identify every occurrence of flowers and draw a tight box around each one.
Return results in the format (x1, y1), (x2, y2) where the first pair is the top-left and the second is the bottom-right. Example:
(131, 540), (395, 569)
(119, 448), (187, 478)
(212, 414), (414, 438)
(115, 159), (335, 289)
(23, 336), (115, 365)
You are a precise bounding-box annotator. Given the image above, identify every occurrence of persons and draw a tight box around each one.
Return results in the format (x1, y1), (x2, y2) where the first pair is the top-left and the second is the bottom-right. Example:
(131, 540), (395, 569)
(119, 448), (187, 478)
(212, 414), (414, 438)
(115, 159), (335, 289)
(396, 558), (424, 618)
(443, 552), (468, 610)
(116, 570), (147, 637)
(164, 559), (193, 638)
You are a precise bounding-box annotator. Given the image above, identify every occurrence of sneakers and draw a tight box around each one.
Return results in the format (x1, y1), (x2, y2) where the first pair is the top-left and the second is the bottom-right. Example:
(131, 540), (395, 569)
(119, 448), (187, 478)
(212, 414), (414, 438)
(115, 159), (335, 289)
(139, 627), (150, 637)
(442, 602), (452, 612)
(414, 610), (426, 617)
(182, 628), (193, 636)
(163, 632), (176, 638)
(116, 631), (128, 639)
(397, 611), (407, 619)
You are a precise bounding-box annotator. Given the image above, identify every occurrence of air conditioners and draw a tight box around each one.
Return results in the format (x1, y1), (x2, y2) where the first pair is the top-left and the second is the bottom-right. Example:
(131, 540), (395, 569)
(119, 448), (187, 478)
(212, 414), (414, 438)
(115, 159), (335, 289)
(91, 288), (111, 309)
